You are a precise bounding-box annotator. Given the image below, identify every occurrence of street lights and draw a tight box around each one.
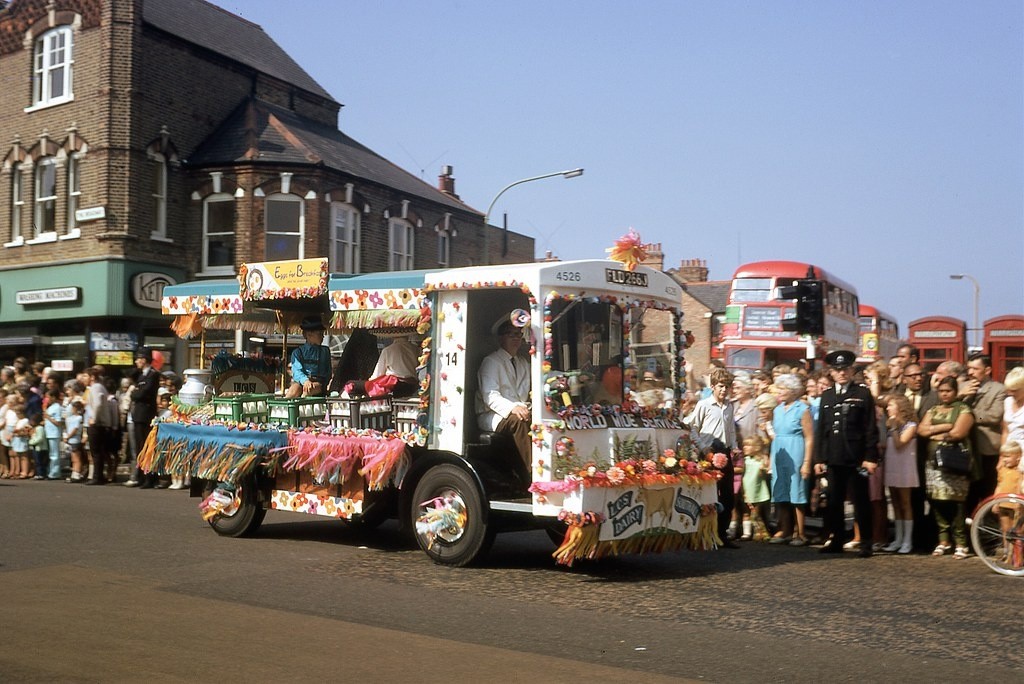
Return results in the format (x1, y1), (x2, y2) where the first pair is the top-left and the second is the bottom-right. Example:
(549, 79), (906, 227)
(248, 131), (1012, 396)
(484, 167), (586, 265)
(949, 273), (980, 347)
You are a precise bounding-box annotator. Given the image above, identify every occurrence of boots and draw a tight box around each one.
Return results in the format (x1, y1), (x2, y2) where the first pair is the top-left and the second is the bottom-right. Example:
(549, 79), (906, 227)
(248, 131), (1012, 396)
(726, 521), (738, 540)
(898, 520), (914, 554)
(741, 521), (752, 540)
(884, 520), (903, 552)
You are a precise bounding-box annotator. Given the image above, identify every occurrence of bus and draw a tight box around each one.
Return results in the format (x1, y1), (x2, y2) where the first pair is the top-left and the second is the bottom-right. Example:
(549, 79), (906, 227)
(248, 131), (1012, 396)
(723, 261), (862, 377)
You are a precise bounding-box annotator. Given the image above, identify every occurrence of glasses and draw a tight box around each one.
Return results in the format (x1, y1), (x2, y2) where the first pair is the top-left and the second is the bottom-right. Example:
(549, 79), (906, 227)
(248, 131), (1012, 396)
(501, 330), (523, 338)
(903, 372), (927, 379)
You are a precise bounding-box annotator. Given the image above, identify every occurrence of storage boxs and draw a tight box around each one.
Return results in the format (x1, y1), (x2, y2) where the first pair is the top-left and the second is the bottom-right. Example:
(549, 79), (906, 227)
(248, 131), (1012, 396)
(212, 392), (275, 424)
(266, 395), (327, 429)
(324, 395), (395, 433)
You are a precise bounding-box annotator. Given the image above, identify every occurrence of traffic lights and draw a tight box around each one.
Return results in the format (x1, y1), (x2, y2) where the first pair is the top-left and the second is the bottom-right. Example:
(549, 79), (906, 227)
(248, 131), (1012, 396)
(778, 281), (822, 335)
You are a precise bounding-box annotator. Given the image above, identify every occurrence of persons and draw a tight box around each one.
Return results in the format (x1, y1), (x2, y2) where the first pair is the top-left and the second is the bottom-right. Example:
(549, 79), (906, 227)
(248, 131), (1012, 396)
(0, 292), (1023, 569)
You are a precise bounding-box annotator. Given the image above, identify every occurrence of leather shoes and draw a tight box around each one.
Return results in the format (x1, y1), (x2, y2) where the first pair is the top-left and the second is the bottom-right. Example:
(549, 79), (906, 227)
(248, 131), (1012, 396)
(0, 465), (186, 489)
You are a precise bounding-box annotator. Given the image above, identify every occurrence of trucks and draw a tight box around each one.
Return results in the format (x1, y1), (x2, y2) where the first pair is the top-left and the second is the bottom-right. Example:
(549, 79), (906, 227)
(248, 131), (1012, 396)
(148, 257), (718, 567)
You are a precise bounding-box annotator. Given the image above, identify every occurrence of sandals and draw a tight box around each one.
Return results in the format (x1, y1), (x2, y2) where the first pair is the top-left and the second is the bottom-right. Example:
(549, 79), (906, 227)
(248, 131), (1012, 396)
(954, 547), (969, 559)
(932, 545), (952, 555)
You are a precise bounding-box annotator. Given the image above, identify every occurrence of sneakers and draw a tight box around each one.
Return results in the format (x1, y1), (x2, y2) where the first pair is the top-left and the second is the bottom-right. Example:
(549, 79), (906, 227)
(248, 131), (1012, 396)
(752, 531), (885, 557)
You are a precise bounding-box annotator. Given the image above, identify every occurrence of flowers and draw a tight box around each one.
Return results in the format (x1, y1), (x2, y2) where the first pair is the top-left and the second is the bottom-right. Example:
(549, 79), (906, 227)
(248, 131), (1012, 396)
(569, 435), (732, 486)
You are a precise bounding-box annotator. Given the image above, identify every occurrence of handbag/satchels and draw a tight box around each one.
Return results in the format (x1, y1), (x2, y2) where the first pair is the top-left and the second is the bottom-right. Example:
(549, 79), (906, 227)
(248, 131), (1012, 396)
(934, 439), (975, 476)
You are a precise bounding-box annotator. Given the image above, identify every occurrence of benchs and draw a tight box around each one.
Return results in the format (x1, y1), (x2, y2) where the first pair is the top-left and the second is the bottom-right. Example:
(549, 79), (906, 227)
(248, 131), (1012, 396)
(465, 425), (514, 452)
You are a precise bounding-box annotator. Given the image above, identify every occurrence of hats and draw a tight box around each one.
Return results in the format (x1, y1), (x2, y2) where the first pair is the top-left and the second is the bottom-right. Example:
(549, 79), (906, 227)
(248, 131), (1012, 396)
(824, 350), (857, 369)
(492, 313), (511, 337)
(133, 346), (152, 360)
(300, 316), (327, 331)
